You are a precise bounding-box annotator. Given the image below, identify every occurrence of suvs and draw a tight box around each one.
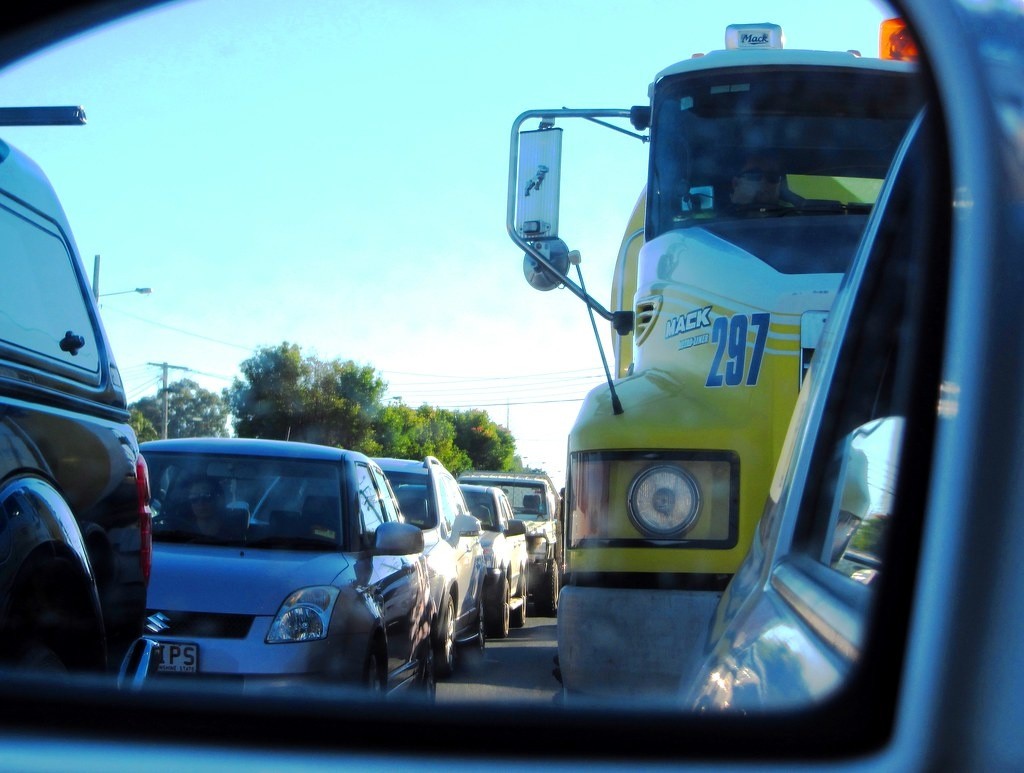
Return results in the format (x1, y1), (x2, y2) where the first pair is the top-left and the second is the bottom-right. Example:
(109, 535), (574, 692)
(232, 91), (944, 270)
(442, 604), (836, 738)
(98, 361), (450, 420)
(460, 472), (567, 616)
(290, 457), (491, 684)
(0, 145), (156, 680)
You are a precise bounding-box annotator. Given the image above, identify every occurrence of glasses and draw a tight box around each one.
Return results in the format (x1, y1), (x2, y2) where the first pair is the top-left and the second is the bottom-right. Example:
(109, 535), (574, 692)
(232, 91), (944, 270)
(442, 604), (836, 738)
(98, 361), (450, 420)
(187, 492), (215, 504)
(736, 167), (785, 185)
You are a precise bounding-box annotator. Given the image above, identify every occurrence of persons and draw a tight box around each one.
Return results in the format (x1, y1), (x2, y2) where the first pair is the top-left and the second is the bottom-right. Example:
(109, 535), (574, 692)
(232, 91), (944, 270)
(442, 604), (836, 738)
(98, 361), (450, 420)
(720, 159), (782, 211)
(162, 476), (247, 538)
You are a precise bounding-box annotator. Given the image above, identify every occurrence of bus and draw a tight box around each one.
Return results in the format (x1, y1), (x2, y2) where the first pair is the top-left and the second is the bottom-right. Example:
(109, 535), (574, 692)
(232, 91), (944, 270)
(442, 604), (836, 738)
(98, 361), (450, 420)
(503, 21), (920, 691)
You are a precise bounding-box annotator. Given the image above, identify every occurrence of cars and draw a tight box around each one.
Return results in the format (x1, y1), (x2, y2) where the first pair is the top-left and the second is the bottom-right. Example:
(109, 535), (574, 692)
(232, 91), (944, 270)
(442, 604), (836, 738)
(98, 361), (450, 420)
(391, 482), (533, 636)
(132, 437), (441, 708)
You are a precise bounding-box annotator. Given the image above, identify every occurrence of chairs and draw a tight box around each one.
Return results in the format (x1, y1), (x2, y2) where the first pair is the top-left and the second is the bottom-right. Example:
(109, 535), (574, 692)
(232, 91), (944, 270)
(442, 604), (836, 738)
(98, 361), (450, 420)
(403, 497), (428, 518)
(523, 495), (540, 510)
(301, 495), (339, 535)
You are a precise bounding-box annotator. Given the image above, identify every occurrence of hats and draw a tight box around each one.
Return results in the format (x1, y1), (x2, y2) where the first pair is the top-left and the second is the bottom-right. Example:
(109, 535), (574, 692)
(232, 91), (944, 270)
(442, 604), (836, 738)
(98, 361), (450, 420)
(732, 151), (769, 174)
